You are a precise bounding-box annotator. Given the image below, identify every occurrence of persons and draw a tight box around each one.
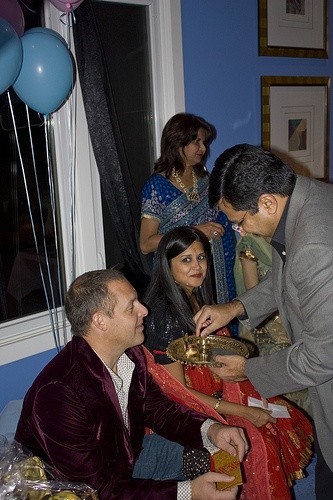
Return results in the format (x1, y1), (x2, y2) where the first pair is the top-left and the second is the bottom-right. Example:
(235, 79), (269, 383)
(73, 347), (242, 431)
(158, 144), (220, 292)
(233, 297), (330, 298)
(139, 112), (239, 339)
(193, 143), (333, 474)
(13, 268), (248, 500)
(141, 226), (313, 500)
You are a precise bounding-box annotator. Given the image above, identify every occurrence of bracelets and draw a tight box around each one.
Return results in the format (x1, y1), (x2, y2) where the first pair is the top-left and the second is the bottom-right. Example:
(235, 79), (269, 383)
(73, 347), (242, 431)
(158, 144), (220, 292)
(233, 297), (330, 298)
(214, 398), (222, 411)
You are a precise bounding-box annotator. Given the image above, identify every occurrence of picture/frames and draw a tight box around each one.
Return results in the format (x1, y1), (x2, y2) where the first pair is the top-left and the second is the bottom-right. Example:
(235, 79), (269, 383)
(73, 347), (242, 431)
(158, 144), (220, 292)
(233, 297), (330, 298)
(261, 75), (331, 183)
(258, 0), (329, 59)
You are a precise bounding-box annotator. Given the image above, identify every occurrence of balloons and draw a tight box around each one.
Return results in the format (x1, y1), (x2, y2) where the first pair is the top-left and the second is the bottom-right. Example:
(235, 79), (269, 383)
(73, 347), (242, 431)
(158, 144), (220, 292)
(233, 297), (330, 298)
(0, 0), (85, 117)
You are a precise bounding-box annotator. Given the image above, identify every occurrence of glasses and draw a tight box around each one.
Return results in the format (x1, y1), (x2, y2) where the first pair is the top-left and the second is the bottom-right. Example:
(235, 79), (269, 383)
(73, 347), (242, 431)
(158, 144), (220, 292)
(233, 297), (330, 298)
(232, 204), (250, 233)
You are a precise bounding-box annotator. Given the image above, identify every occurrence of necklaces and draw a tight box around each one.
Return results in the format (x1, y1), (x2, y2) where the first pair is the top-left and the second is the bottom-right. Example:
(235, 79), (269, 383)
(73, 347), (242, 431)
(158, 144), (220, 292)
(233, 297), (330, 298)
(172, 167), (199, 202)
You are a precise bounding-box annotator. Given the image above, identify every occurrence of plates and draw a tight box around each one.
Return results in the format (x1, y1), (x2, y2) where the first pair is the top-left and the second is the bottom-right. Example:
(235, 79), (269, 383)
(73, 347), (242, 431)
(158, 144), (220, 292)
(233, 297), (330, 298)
(166, 334), (251, 367)
